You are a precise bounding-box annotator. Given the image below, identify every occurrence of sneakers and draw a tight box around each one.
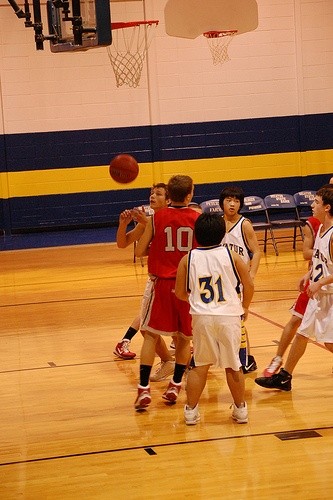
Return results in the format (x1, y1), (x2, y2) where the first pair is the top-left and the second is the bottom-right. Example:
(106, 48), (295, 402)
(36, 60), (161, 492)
(254, 369), (292, 391)
(231, 401), (249, 423)
(184, 403), (201, 425)
(134, 385), (152, 410)
(166, 342), (195, 356)
(263, 356), (282, 377)
(150, 358), (175, 382)
(113, 338), (137, 359)
(161, 381), (182, 403)
(243, 355), (257, 373)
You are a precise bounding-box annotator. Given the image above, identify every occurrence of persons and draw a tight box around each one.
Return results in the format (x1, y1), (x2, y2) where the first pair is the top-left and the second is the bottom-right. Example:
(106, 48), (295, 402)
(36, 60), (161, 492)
(262, 176), (333, 377)
(254, 187), (333, 392)
(112, 175), (262, 425)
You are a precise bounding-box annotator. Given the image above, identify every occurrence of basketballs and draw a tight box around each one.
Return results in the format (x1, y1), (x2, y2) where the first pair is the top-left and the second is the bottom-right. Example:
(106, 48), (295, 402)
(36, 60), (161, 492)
(109, 153), (139, 183)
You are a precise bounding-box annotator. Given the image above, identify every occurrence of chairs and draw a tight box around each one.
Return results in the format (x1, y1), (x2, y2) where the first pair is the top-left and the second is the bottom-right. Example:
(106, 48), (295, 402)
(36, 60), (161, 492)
(133, 190), (316, 268)
